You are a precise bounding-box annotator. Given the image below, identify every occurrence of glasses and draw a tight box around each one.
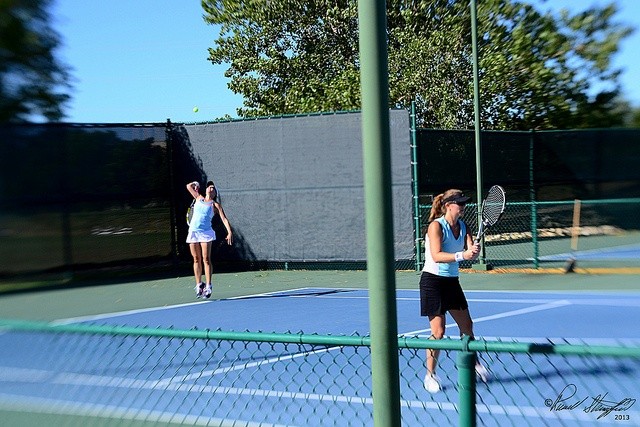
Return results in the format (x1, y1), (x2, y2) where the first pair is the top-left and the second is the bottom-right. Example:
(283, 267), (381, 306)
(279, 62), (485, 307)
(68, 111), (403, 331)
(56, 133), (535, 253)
(449, 202), (466, 206)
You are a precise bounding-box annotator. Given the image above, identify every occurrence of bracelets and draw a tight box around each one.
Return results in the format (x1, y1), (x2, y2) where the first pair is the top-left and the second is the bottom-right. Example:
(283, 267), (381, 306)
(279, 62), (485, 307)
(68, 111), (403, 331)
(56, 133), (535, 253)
(455, 251), (464, 262)
(192, 182), (199, 186)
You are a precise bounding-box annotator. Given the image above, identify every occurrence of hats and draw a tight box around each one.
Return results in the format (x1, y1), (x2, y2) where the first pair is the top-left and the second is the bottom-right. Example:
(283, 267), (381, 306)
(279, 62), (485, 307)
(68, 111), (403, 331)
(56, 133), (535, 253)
(206, 180), (217, 192)
(439, 194), (471, 203)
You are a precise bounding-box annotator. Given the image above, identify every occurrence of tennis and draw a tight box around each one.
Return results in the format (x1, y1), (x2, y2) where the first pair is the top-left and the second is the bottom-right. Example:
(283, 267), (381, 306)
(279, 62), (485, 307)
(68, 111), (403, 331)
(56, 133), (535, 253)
(193, 107), (199, 112)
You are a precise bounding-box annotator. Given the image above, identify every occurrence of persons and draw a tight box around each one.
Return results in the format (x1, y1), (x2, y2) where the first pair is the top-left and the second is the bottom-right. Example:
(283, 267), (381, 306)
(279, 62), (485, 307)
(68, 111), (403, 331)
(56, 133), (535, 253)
(419, 190), (488, 392)
(186, 181), (233, 298)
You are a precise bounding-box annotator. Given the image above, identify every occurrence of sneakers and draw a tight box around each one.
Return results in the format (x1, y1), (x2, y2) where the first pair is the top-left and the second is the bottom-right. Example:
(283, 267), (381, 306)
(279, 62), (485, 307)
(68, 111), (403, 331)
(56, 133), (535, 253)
(194, 282), (205, 298)
(203, 285), (212, 299)
(474, 358), (488, 383)
(423, 372), (442, 393)
